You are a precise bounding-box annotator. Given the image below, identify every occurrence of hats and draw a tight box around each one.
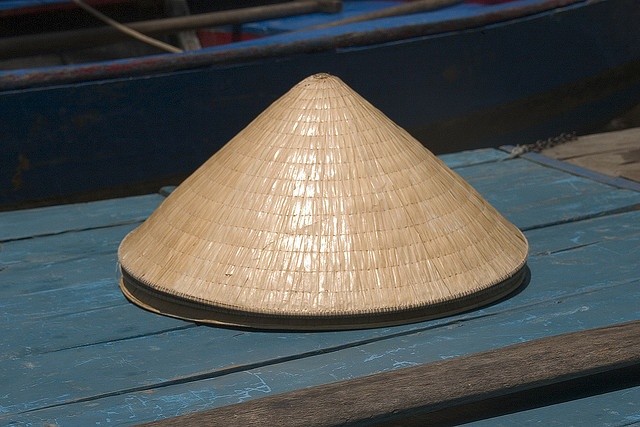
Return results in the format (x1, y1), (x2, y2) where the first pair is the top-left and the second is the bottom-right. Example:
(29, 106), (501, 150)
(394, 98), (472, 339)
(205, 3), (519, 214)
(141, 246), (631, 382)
(118, 74), (530, 332)
